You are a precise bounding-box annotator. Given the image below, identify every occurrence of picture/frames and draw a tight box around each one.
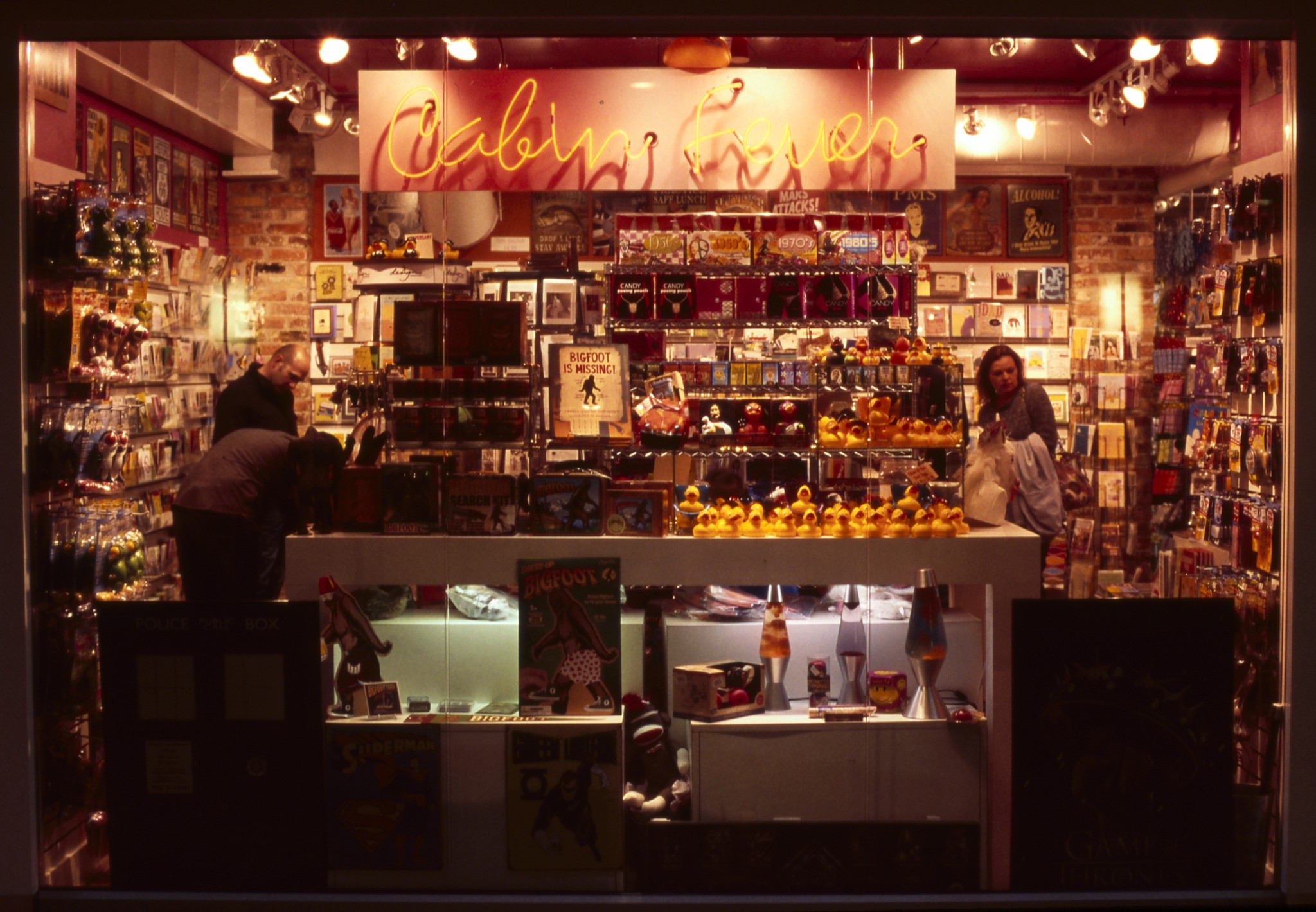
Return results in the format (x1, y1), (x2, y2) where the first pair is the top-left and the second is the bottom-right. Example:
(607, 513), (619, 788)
(940, 172), (1070, 262)
(549, 338), (634, 441)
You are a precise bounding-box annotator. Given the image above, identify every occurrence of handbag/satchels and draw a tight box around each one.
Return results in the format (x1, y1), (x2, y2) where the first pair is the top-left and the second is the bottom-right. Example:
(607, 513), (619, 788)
(1050, 445), (1096, 509)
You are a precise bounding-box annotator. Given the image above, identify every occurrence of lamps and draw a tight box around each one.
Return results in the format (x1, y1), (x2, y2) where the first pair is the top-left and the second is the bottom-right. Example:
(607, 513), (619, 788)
(949, 36), (1223, 136)
(659, 36), (733, 76)
(231, 34), (481, 140)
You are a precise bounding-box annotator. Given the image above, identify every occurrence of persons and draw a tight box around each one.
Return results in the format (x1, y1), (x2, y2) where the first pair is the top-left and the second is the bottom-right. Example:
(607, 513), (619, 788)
(978, 344), (1060, 599)
(702, 404), (732, 435)
(213, 345), (311, 599)
(173, 422), (343, 597)
